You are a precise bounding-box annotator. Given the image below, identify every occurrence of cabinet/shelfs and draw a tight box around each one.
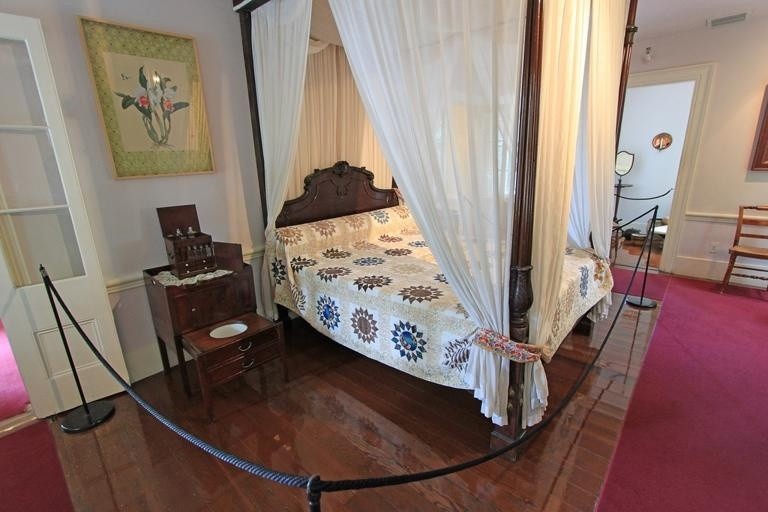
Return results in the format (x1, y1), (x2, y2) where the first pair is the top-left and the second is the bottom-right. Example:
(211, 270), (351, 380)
(142, 263), (293, 424)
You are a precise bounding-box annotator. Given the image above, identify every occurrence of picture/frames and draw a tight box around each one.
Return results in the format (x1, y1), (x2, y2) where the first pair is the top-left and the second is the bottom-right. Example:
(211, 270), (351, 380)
(79, 14), (217, 179)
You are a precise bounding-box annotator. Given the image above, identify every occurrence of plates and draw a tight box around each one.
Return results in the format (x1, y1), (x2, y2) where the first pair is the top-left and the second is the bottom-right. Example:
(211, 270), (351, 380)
(209, 322), (248, 340)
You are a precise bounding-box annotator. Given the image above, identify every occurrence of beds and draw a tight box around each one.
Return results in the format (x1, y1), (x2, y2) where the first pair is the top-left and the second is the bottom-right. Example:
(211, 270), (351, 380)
(278, 161), (596, 465)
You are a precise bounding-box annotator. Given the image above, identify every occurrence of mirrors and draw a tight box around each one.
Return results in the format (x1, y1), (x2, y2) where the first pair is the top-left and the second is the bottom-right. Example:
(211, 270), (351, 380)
(615, 151), (635, 176)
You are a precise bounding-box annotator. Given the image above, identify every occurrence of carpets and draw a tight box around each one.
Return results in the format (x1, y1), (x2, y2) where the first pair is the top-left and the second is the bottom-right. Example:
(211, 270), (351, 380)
(2, 418), (75, 512)
(595, 264), (766, 511)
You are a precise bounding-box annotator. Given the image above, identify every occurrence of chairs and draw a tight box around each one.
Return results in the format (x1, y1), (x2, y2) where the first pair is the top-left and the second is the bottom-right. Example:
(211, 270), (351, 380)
(718, 205), (767, 296)
